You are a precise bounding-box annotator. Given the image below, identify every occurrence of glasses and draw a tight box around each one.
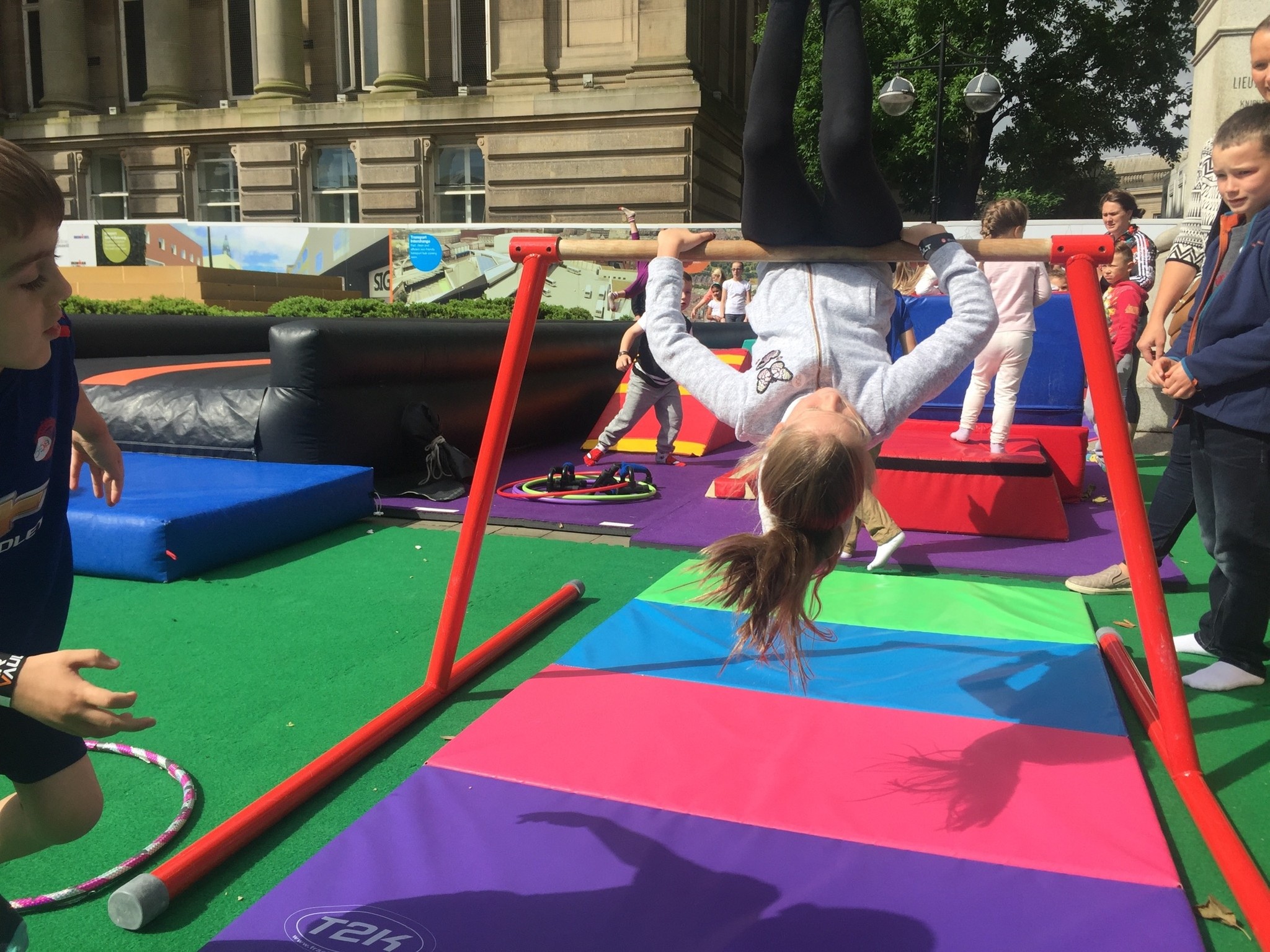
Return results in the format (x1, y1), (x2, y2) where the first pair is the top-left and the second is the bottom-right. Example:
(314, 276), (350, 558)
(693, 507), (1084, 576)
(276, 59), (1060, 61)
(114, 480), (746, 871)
(732, 268), (741, 271)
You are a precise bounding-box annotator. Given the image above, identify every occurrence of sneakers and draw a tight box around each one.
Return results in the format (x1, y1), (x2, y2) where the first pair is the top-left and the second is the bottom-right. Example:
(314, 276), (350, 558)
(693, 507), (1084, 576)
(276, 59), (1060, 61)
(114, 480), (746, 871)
(1065, 564), (1131, 595)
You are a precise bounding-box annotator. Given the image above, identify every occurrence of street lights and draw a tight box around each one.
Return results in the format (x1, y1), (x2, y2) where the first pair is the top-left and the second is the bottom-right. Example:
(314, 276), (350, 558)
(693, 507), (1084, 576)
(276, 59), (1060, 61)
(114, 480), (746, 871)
(876, 9), (1006, 225)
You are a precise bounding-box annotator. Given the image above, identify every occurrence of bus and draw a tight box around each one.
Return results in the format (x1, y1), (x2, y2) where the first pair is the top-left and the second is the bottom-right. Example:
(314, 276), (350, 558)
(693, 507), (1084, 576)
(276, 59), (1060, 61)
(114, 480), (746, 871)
(598, 285), (605, 300)
(594, 300), (603, 318)
(567, 266), (582, 275)
(545, 276), (556, 287)
(542, 287), (551, 297)
(584, 284), (591, 298)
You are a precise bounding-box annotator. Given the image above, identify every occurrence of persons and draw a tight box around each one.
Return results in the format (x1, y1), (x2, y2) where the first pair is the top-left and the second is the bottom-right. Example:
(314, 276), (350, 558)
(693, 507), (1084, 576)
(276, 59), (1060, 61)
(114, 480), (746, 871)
(0, 140), (157, 952)
(583, 0), (1269, 695)
